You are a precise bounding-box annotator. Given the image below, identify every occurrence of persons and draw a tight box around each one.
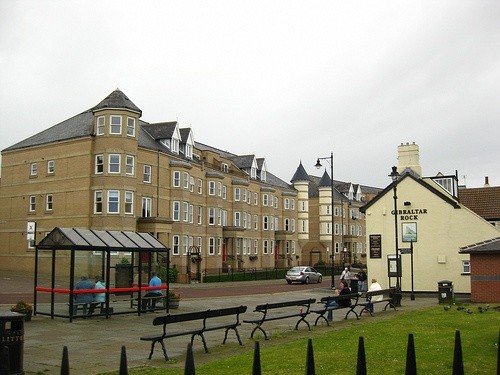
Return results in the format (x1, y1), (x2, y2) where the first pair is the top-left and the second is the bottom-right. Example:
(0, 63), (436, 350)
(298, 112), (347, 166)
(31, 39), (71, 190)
(142, 272), (161, 314)
(87, 275), (106, 317)
(365, 278), (383, 313)
(324, 280), (351, 322)
(357, 266), (367, 291)
(71, 273), (94, 318)
(340, 268), (350, 287)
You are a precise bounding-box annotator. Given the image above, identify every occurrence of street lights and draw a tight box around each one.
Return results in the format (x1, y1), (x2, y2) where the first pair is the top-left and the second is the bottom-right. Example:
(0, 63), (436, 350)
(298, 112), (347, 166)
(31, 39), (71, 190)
(388, 165), (400, 287)
(313, 152), (335, 290)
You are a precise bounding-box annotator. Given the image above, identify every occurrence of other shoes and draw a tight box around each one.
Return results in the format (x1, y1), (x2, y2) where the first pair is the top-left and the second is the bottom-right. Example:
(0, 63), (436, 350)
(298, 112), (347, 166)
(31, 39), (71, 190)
(363, 310), (367, 313)
(327, 318), (332, 321)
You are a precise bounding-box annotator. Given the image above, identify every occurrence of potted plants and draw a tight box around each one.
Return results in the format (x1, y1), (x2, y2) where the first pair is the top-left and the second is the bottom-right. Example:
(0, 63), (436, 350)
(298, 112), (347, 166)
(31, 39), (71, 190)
(162, 291), (180, 308)
(9, 301), (32, 322)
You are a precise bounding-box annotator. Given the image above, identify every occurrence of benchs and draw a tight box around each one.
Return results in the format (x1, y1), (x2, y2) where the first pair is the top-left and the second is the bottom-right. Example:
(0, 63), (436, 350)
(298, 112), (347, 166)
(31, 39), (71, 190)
(129, 296), (165, 311)
(309, 293), (359, 327)
(140, 304), (247, 361)
(359, 288), (398, 317)
(242, 298), (316, 340)
(65, 301), (105, 319)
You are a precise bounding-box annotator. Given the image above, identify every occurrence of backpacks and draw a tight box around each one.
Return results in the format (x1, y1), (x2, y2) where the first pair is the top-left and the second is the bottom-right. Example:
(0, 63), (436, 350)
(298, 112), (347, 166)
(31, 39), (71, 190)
(359, 271), (367, 281)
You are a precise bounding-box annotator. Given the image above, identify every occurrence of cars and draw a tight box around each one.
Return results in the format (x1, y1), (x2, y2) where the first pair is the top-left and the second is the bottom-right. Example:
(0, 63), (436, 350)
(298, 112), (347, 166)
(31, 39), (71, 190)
(285, 265), (322, 285)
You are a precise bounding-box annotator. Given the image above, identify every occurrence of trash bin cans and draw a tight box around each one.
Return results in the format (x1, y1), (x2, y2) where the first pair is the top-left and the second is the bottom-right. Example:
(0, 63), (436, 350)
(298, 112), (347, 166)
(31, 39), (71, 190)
(0, 311), (26, 375)
(438, 280), (454, 304)
